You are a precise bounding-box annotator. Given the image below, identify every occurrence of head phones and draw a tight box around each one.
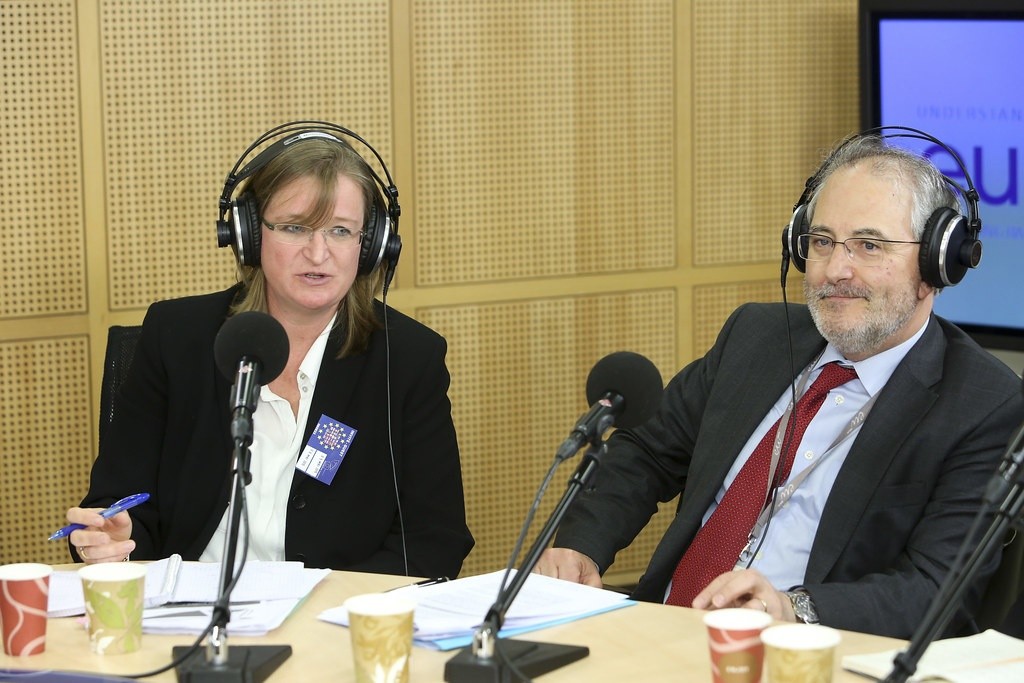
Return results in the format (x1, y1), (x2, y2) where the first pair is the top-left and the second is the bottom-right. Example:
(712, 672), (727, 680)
(788, 127), (982, 289)
(216, 120), (403, 277)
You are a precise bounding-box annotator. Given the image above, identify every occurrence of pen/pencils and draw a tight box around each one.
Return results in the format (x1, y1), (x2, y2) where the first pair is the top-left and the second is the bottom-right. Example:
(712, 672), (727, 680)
(383, 576), (449, 594)
(46, 492), (150, 541)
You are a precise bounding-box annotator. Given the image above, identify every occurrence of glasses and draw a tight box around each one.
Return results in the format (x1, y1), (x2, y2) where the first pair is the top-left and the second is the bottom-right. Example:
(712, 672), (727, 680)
(259, 216), (368, 248)
(797, 234), (921, 268)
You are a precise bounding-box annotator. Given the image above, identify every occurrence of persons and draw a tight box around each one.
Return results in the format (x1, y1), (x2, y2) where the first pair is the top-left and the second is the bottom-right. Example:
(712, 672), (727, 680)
(67, 120), (476, 581)
(533, 124), (1024, 641)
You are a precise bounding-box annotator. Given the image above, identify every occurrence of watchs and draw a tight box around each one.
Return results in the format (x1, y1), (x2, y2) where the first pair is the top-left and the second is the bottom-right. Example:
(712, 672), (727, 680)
(784, 590), (820, 625)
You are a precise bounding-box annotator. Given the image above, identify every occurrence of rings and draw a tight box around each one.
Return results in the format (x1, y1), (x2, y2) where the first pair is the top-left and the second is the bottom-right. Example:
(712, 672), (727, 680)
(79, 547), (88, 558)
(761, 599), (767, 612)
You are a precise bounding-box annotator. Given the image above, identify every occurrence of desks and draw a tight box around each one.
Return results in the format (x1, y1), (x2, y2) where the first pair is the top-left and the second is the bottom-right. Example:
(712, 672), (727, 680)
(0, 559), (954, 683)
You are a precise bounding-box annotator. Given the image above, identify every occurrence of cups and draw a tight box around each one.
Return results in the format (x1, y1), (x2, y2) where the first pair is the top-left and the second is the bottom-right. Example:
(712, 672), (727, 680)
(0, 562), (53, 657)
(760, 624), (843, 683)
(703, 607), (772, 683)
(342, 592), (419, 683)
(76, 561), (147, 655)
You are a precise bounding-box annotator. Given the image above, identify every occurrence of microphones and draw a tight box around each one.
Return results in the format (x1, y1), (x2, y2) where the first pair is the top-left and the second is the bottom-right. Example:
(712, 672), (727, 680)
(557, 351), (664, 462)
(215, 311), (289, 444)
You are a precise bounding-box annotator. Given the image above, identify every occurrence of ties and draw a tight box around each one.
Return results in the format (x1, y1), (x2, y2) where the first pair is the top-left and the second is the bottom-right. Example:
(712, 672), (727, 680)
(663, 360), (859, 608)
